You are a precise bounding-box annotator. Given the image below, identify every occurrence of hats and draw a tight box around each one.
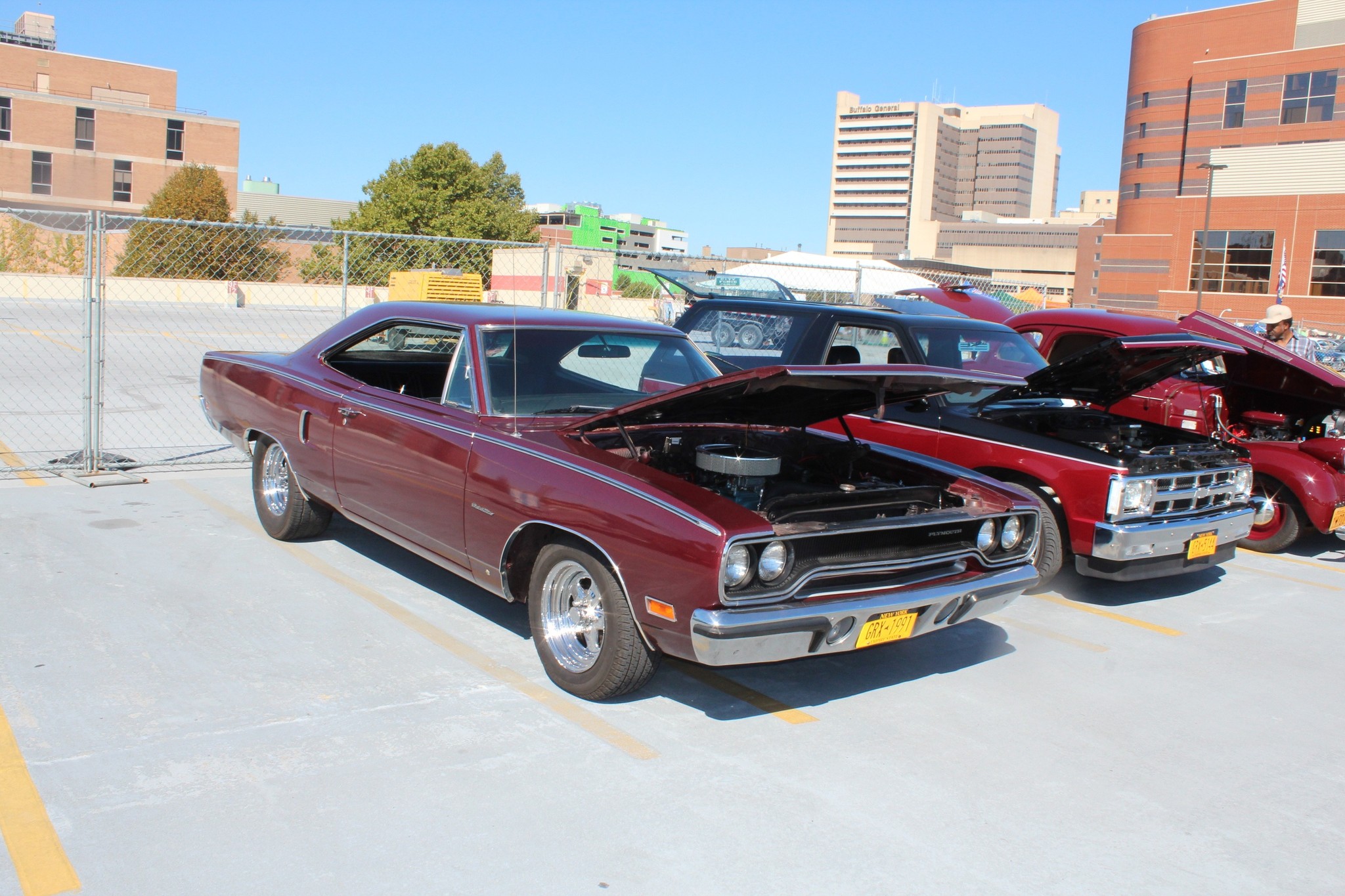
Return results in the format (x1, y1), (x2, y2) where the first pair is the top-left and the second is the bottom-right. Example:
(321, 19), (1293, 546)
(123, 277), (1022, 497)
(1259, 305), (1292, 325)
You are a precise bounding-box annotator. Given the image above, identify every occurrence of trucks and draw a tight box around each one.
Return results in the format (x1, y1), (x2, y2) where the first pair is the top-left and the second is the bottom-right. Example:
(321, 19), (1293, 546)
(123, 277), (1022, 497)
(675, 291), (792, 350)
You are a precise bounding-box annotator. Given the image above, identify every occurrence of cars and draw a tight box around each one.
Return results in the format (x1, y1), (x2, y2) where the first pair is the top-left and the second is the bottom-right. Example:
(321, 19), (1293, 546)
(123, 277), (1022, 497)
(636, 266), (1256, 593)
(896, 284), (1345, 553)
(200, 298), (1043, 703)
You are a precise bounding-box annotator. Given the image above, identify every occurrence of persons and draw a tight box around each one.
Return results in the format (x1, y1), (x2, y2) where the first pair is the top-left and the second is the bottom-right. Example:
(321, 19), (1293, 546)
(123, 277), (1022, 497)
(1260, 306), (1321, 362)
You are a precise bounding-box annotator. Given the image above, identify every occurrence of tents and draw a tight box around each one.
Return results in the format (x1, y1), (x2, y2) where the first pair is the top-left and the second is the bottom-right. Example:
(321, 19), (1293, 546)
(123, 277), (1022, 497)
(990, 287), (1072, 312)
(693, 248), (938, 296)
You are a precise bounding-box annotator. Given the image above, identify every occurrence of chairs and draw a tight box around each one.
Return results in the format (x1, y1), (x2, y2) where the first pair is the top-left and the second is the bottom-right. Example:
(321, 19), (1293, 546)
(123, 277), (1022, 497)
(824, 346), (861, 366)
(887, 346), (909, 364)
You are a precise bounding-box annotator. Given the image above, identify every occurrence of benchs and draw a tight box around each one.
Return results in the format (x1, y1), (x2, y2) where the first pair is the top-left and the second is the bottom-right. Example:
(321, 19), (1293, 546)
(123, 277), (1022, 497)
(329, 361), (515, 399)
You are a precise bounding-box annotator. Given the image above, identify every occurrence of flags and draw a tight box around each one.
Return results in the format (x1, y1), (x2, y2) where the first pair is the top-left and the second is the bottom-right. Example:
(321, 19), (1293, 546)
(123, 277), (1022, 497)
(1277, 246), (1286, 304)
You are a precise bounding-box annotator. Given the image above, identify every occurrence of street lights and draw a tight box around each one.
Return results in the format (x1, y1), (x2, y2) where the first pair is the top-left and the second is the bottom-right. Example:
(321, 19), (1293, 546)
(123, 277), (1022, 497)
(1196, 164), (1228, 310)
